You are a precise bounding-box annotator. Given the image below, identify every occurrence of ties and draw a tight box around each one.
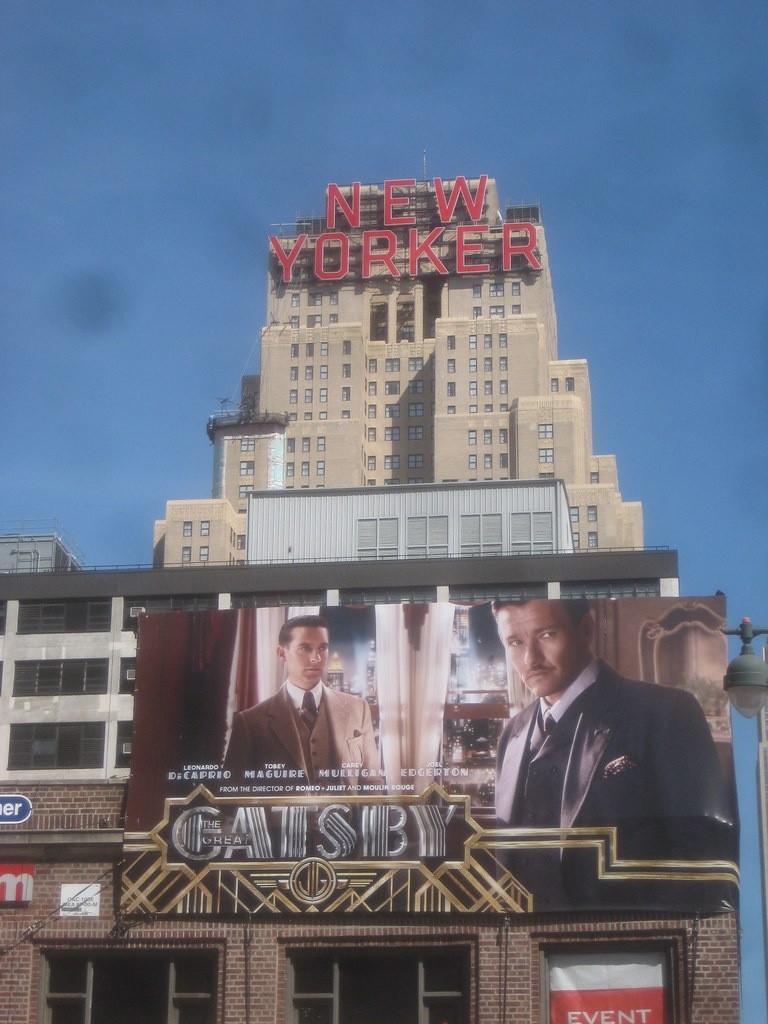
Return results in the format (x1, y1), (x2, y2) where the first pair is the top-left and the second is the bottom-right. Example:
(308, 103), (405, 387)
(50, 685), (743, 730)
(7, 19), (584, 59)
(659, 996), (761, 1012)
(301, 691), (317, 731)
(542, 711), (555, 738)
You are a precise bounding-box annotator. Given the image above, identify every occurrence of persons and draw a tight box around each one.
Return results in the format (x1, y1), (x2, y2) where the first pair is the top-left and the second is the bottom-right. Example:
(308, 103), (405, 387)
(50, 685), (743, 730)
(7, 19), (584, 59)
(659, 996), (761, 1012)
(490, 598), (729, 914)
(222, 613), (383, 797)
